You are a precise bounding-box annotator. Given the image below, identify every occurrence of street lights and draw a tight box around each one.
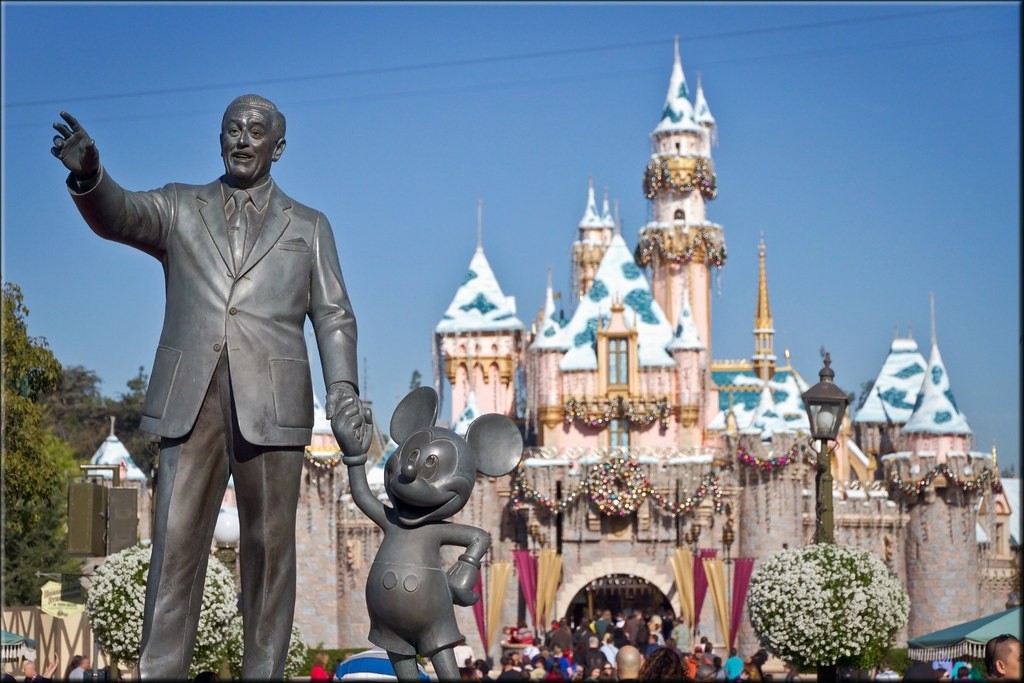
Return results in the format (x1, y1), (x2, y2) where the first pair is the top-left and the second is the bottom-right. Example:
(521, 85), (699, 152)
(795, 351), (849, 683)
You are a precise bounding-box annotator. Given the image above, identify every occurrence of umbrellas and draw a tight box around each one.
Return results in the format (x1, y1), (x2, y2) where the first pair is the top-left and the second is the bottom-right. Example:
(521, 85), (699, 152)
(908, 606), (1019, 661)
(1, 631), (37, 661)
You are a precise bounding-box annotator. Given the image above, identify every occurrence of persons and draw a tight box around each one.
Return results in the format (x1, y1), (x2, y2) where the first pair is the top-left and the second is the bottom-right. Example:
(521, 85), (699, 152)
(875, 665), (898, 680)
(50, 93), (364, 680)
(984, 634), (1021, 680)
(784, 664), (800, 680)
(454, 606), (773, 680)
(903, 659), (972, 680)
(196, 672), (221, 680)
(1, 654), (90, 681)
(310, 653), (353, 680)
